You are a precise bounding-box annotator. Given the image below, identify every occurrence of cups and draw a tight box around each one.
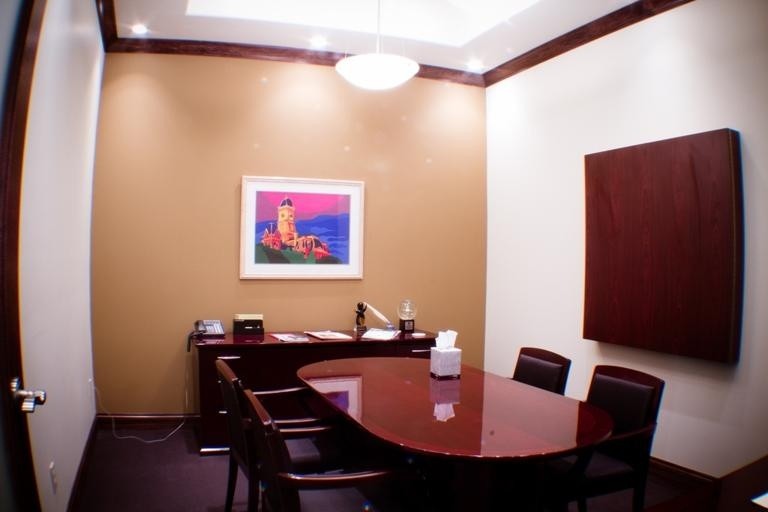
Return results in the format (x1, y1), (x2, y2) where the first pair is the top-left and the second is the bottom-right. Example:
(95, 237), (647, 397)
(399, 301), (415, 320)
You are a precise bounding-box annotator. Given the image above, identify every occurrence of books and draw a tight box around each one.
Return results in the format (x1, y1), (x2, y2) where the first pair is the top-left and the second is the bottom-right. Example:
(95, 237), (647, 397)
(269, 331), (312, 342)
(361, 327), (402, 341)
(303, 330), (353, 341)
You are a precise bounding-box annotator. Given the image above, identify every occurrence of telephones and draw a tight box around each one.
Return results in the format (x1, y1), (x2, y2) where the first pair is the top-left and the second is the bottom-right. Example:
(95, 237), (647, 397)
(194, 320), (225, 338)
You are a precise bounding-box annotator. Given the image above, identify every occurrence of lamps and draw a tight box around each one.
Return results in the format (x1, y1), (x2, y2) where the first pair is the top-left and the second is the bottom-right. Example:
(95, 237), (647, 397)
(335, 3), (419, 94)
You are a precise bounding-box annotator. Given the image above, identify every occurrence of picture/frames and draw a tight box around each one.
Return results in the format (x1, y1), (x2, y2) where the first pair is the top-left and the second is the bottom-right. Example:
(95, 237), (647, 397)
(241, 175), (364, 279)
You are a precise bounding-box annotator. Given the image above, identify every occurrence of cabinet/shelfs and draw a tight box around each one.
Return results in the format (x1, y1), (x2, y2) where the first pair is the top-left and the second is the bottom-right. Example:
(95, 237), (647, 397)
(184, 329), (438, 455)
